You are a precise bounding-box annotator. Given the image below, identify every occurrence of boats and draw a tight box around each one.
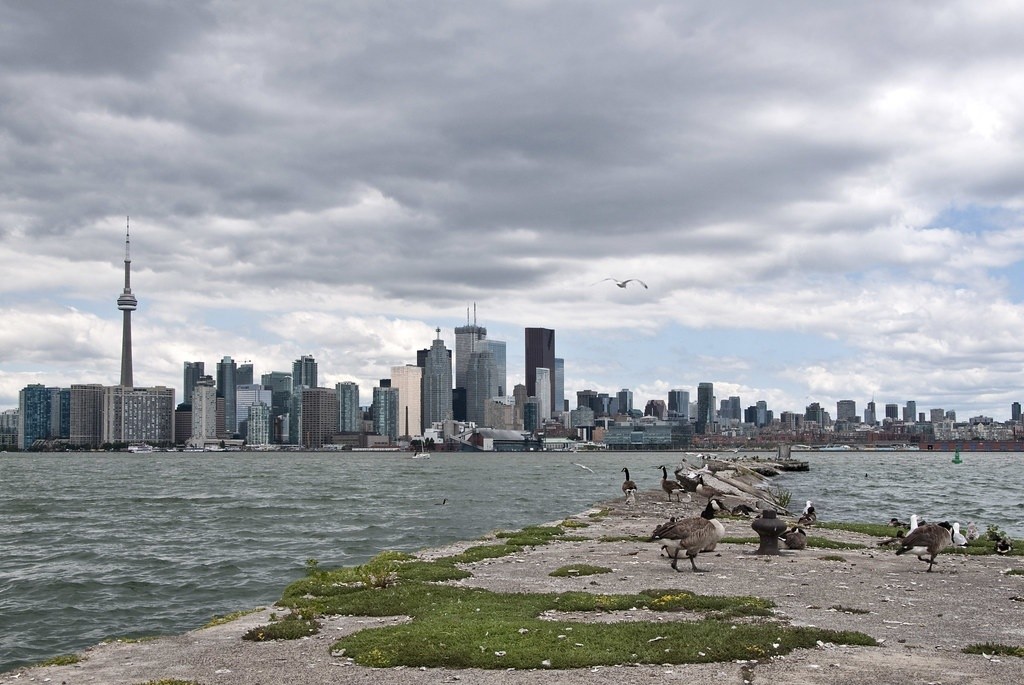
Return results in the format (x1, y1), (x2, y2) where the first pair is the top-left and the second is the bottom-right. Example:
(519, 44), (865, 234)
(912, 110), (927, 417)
(819, 443), (857, 451)
(790, 445), (818, 452)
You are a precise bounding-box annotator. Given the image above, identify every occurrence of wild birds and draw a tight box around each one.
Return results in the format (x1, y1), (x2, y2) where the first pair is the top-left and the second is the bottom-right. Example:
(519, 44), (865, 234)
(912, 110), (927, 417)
(591, 275), (649, 291)
(570, 460), (596, 474)
(433, 497), (449, 506)
(620, 449), (1013, 574)
